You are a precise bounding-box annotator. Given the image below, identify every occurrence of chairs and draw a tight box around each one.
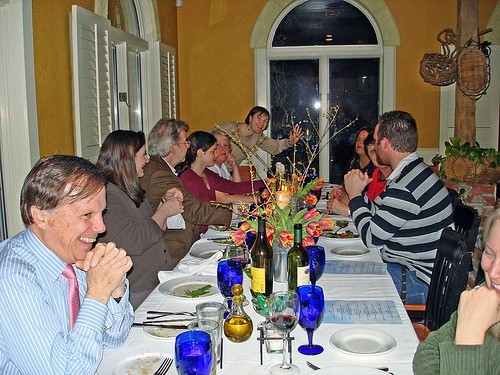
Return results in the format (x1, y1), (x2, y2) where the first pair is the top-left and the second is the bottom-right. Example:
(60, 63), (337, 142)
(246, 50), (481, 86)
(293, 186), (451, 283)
(410, 188), (483, 342)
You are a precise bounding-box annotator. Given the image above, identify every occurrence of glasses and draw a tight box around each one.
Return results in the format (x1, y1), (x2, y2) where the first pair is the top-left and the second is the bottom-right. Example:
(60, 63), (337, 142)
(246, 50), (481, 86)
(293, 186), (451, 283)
(172, 139), (191, 148)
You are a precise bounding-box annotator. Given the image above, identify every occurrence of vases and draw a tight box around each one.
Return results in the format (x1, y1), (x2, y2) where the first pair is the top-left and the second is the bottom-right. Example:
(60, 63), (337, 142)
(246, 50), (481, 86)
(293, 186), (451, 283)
(271, 239), (291, 283)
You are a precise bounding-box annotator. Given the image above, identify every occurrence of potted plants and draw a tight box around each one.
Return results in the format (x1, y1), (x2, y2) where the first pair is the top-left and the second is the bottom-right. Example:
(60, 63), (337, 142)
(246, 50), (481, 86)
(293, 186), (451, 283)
(432, 136), (500, 186)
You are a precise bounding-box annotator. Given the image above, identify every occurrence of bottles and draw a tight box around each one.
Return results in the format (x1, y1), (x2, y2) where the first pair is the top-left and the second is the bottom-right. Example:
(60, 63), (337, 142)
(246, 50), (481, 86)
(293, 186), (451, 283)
(224, 284), (253, 342)
(286, 223), (310, 293)
(249, 216), (272, 297)
(245, 163), (321, 250)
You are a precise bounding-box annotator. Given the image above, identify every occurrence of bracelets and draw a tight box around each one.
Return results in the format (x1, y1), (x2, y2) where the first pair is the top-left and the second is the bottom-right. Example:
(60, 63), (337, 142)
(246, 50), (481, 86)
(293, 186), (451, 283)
(161, 197), (164, 203)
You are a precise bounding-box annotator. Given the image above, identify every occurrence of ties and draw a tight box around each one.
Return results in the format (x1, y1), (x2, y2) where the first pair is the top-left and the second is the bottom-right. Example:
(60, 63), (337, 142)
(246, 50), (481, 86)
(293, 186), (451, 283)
(62, 264), (81, 330)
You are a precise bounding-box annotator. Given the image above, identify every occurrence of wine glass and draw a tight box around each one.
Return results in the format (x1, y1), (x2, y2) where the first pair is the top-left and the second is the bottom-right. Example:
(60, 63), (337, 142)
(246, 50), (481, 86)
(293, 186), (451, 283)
(294, 285), (324, 356)
(268, 292), (300, 374)
(217, 243), (250, 318)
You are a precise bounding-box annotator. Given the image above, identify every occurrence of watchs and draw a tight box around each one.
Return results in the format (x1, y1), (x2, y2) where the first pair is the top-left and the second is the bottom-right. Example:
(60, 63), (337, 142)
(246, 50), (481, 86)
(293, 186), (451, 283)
(347, 208), (351, 218)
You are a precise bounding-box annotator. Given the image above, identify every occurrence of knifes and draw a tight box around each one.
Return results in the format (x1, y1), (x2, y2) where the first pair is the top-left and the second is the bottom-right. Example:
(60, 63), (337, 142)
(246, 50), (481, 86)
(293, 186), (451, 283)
(131, 322), (187, 328)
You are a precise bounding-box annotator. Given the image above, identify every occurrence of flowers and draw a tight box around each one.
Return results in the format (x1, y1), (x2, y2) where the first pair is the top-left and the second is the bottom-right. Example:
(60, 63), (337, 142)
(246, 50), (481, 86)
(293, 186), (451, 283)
(210, 104), (360, 250)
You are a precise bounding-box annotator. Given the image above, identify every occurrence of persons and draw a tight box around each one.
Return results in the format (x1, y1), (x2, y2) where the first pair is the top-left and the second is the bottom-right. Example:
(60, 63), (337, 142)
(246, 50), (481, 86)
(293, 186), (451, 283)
(0, 154), (134, 375)
(209, 106), (304, 176)
(95, 129), (184, 311)
(412, 207), (500, 375)
(326, 110), (456, 305)
(174, 130), (270, 235)
(206, 131), (261, 204)
(135, 118), (249, 269)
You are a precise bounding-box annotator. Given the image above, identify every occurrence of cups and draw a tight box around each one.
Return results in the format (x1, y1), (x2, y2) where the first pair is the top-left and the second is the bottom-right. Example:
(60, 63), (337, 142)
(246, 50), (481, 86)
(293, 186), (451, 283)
(175, 301), (224, 375)
(305, 245), (326, 284)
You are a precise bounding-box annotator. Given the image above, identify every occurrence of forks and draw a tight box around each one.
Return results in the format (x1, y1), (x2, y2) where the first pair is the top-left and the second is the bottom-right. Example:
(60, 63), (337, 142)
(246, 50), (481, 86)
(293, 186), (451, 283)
(154, 358), (173, 375)
(307, 361), (389, 372)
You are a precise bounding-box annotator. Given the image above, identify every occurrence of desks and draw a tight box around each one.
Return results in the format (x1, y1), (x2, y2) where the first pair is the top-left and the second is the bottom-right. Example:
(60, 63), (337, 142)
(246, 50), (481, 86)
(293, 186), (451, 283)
(95, 184), (420, 375)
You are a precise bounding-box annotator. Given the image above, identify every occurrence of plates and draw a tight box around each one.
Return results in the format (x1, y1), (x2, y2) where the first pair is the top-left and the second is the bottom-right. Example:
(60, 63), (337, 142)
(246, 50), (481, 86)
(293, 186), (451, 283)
(159, 274), (220, 299)
(321, 227), (362, 240)
(190, 245), (226, 260)
(322, 183), (342, 193)
(330, 245), (371, 258)
(309, 328), (397, 375)
(143, 305), (198, 339)
(97, 342), (178, 375)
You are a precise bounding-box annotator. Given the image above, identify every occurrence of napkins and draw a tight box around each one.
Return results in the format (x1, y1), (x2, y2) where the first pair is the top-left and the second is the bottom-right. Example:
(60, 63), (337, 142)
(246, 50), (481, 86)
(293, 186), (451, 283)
(176, 260), (218, 276)
(157, 251), (225, 285)
